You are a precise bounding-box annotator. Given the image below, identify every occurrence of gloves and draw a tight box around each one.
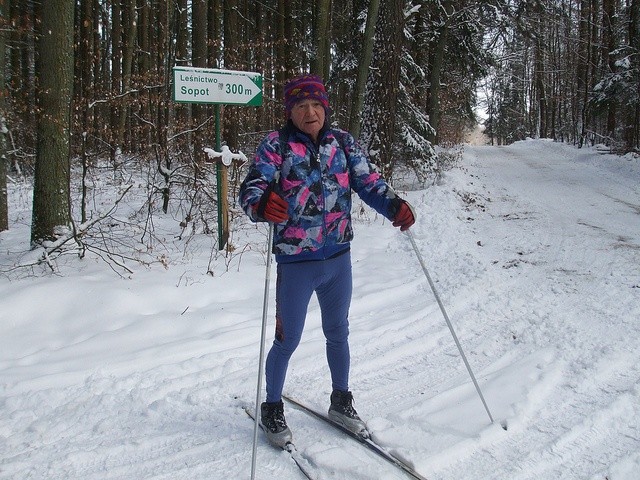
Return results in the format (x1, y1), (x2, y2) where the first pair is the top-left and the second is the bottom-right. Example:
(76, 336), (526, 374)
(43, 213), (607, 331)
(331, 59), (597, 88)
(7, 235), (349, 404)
(391, 198), (416, 232)
(252, 188), (291, 223)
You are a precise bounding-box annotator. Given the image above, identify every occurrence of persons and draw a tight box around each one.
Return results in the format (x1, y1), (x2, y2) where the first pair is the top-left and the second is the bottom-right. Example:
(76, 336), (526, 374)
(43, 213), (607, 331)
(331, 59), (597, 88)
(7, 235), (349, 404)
(237, 74), (417, 446)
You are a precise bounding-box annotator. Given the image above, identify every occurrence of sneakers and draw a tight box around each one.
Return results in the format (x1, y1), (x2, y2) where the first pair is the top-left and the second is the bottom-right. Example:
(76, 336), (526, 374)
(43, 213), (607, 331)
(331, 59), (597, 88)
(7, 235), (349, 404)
(328, 390), (371, 438)
(261, 400), (291, 446)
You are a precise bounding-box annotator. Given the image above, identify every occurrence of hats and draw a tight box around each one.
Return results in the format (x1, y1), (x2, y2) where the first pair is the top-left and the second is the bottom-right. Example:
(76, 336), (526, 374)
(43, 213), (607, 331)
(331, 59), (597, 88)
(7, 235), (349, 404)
(283, 75), (331, 123)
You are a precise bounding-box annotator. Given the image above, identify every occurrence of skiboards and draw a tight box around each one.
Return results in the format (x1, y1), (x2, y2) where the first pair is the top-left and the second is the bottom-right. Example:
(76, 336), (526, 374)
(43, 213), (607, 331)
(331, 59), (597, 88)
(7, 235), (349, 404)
(239, 389), (427, 480)
